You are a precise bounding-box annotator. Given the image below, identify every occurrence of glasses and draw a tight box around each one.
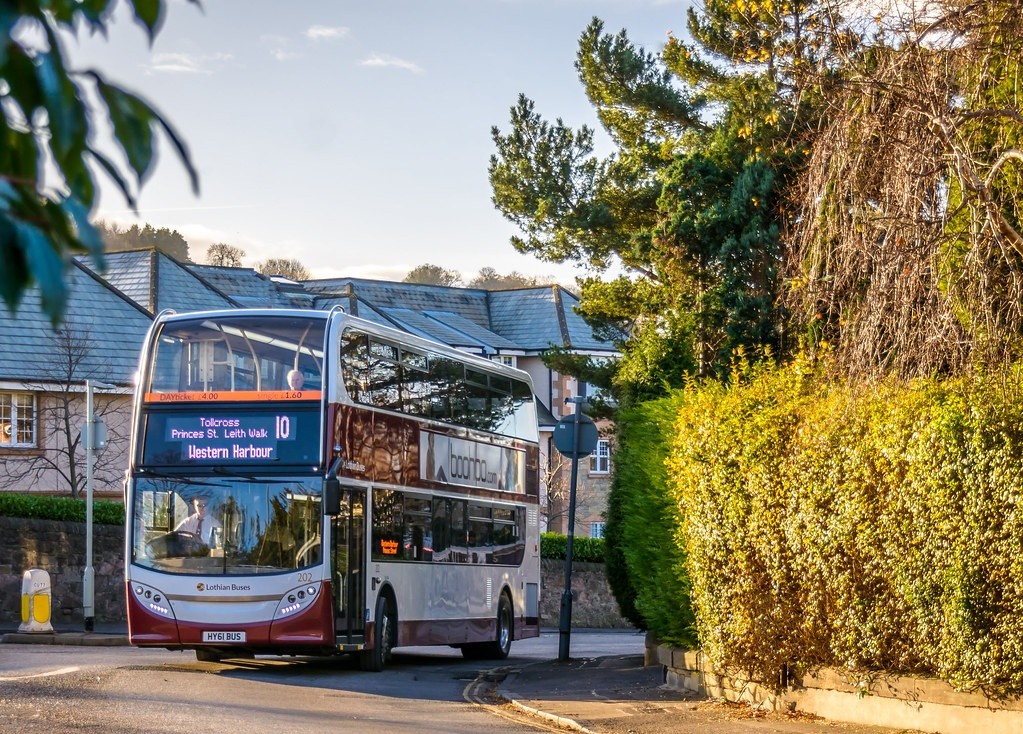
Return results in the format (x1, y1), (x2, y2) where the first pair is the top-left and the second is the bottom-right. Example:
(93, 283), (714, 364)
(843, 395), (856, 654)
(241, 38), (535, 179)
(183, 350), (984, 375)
(195, 502), (209, 507)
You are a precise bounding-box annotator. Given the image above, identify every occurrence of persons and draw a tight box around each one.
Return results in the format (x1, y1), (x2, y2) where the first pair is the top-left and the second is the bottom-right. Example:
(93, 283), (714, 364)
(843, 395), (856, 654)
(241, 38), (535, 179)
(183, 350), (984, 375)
(425, 431), (449, 483)
(499, 446), (518, 492)
(168, 494), (227, 557)
(286, 369), (308, 391)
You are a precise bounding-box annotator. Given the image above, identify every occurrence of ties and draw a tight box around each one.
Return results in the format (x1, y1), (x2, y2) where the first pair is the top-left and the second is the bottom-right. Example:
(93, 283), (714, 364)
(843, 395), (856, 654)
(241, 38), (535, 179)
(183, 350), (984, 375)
(195, 518), (203, 537)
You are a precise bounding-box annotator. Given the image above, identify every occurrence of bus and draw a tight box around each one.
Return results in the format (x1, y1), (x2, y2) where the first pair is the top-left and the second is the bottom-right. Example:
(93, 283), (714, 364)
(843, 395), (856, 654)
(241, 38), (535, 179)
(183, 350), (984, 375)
(119, 305), (542, 676)
